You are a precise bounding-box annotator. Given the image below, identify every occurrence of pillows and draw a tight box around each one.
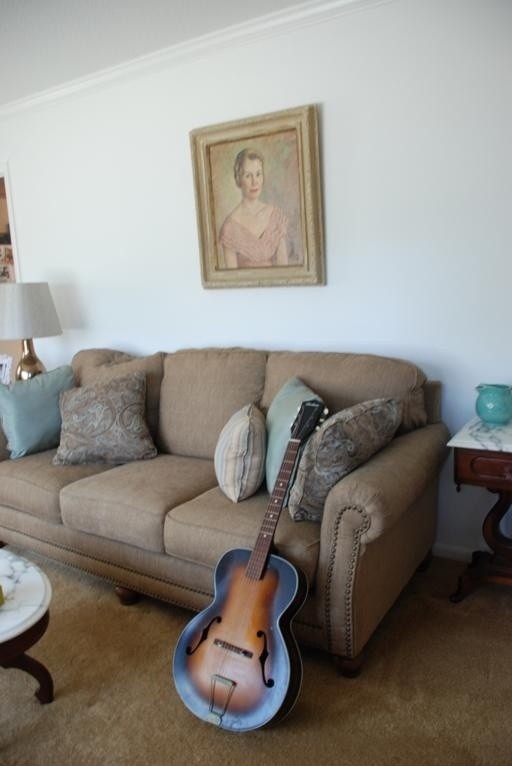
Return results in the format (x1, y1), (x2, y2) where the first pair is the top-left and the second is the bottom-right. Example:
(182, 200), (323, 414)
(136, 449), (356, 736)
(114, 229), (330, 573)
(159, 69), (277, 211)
(286, 394), (407, 522)
(262, 374), (325, 510)
(1, 365), (80, 461)
(52, 369), (159, 467)
(213, 400), (270, 504)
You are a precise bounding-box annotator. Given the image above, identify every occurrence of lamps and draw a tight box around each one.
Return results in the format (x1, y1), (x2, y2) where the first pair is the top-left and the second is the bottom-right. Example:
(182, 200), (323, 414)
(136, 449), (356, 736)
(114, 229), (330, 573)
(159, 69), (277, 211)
(0, 283), (63, 380)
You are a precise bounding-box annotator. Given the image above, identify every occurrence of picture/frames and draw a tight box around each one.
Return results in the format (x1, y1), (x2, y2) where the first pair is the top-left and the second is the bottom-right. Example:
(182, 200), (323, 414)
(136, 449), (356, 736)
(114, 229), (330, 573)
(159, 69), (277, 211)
(0, 174), (20, 283)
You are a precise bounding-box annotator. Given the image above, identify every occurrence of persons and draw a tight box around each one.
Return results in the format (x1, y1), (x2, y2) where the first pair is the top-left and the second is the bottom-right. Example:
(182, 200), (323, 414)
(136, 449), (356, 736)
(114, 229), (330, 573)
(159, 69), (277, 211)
(217, 147), (293, 270)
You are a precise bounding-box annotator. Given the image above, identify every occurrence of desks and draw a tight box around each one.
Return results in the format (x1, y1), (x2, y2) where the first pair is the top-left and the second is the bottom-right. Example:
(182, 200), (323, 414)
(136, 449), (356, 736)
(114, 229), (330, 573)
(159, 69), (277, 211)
(445, 415), (512, 604)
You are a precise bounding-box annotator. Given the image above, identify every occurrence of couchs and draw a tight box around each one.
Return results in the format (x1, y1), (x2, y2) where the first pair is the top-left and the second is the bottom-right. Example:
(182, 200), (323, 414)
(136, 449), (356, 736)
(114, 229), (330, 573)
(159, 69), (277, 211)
(0, 346), (450, 672)
(189, 104), (326, 289)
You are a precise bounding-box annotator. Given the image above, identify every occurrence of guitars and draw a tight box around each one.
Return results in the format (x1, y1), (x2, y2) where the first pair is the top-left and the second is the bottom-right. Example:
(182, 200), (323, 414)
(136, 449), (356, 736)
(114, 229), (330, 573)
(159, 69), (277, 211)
(172, 400), (329, 731)
